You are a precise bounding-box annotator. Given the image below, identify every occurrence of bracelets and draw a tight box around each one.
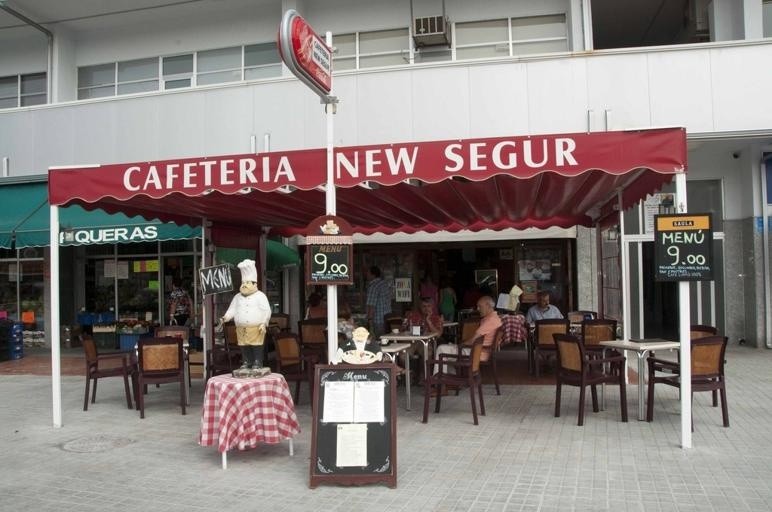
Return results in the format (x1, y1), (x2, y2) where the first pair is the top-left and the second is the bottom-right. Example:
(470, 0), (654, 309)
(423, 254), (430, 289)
(366, 318), (372, 322)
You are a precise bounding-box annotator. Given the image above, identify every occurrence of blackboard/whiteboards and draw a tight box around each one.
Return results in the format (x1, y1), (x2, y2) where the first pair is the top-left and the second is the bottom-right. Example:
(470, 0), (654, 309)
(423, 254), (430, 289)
(310, 363), (397, 489)
(199, 262), (234, 296)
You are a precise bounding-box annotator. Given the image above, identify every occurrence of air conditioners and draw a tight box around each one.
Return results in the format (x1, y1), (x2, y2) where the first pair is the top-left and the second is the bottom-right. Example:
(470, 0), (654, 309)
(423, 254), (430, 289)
(411, 13), (452, 51)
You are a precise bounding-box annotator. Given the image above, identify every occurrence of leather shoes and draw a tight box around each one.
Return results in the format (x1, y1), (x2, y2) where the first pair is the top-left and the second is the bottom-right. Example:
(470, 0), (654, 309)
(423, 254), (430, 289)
(424, 386), (450, 398)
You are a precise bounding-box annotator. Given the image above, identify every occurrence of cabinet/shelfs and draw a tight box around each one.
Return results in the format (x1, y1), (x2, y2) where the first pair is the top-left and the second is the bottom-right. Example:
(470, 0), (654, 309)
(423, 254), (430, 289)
(1, 320), (25, 360)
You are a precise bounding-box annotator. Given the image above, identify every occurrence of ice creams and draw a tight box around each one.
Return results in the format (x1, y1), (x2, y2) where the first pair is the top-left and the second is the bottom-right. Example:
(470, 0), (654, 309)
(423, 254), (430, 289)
(353, 327), (369, 350)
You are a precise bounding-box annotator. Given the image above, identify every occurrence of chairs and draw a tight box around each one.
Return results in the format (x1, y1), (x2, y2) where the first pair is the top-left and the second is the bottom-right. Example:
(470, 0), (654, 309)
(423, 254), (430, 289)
(138, 338), (186, 419)
(115, 320), (153, 395)
(156, 326), (191, 387)
(525, 310), (617, 381)
(82, 335), (138, 411)
(655, 325), (720, 407)
(552, 333), (628, 426)
(423, 335), (485, 425)
(647, 336), (729, 432)
(206, 303), (525, 410)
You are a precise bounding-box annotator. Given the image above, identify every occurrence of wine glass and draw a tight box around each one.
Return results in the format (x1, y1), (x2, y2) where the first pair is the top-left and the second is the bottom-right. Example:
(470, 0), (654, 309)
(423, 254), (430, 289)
(353, 337), (367, 357)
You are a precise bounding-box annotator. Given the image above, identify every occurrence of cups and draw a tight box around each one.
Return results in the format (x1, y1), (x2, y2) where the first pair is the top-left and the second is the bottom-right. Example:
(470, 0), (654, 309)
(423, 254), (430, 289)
(392, 329), (399, 333)
(381, 338), (389, 345)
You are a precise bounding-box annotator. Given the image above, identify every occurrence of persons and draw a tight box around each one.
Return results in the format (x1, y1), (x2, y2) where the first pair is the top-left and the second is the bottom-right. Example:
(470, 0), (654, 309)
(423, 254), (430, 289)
(168, 280), (194, 326)
(437, 278), (457, 321)
(337, 326), (383, 365)
(421, 295), (504, 397)
(217, 258), (271, 370)
(338, 296), (413, 381)
(420, 275), (440, 314)
(366, 265), (393, 341)
(397, 297), (444, 388)
(525, 292), (576, 379)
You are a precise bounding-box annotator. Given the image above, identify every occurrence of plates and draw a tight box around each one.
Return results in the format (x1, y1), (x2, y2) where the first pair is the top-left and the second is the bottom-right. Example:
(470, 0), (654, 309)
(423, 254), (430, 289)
(343, 350), (378, 365)
(379, 343), (390, 346)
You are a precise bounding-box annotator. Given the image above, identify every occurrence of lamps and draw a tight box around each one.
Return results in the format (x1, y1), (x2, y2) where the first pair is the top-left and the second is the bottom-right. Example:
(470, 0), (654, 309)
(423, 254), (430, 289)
(658, 195), (674, 214)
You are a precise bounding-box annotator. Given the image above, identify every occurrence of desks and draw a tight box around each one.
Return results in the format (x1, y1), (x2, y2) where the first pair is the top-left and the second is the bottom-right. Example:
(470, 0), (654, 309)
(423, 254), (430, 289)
(600, 340), (680, 421)
(197, 370), (303, 470)
(134, 341), (190, 405)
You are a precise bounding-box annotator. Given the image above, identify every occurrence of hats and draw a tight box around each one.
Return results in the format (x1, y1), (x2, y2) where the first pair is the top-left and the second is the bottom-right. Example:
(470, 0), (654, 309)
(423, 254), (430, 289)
(237, 259), (259, 283)
